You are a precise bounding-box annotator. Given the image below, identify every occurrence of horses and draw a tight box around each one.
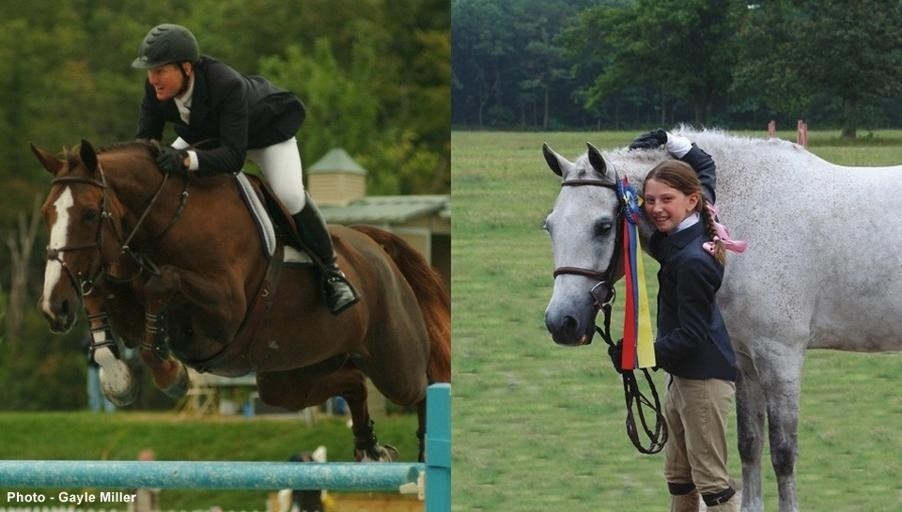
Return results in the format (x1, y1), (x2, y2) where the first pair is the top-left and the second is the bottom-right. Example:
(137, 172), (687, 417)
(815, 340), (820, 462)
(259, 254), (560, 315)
(542, 120), (902, 512)
(29, 139), (451, 463)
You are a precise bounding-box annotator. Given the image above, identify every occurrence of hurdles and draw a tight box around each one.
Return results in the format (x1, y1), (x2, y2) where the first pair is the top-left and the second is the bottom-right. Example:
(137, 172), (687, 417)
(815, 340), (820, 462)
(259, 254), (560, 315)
(2, 381), (452, 510)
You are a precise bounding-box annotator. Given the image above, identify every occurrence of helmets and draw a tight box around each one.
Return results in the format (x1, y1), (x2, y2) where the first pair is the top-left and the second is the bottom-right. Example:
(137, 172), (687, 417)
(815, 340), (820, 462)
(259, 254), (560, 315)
(131, 24), (200, 69)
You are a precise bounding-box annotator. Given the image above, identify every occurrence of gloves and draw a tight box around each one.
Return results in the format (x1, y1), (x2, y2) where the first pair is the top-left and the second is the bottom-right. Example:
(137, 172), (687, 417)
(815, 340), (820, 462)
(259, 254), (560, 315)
(629, 128), (667, 149)
(151, 139), (190, 173)
(608, 339), (623, 374)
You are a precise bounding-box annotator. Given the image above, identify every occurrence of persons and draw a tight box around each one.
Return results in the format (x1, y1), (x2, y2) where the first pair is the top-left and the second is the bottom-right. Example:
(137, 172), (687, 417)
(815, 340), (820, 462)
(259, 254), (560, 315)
(628, 128), (741, 512)
(135, 24), (362, 314)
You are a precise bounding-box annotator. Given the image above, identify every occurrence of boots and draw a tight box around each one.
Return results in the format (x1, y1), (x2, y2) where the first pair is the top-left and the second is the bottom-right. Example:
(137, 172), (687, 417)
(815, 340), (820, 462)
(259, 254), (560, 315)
(290, 191), (361, 314)
